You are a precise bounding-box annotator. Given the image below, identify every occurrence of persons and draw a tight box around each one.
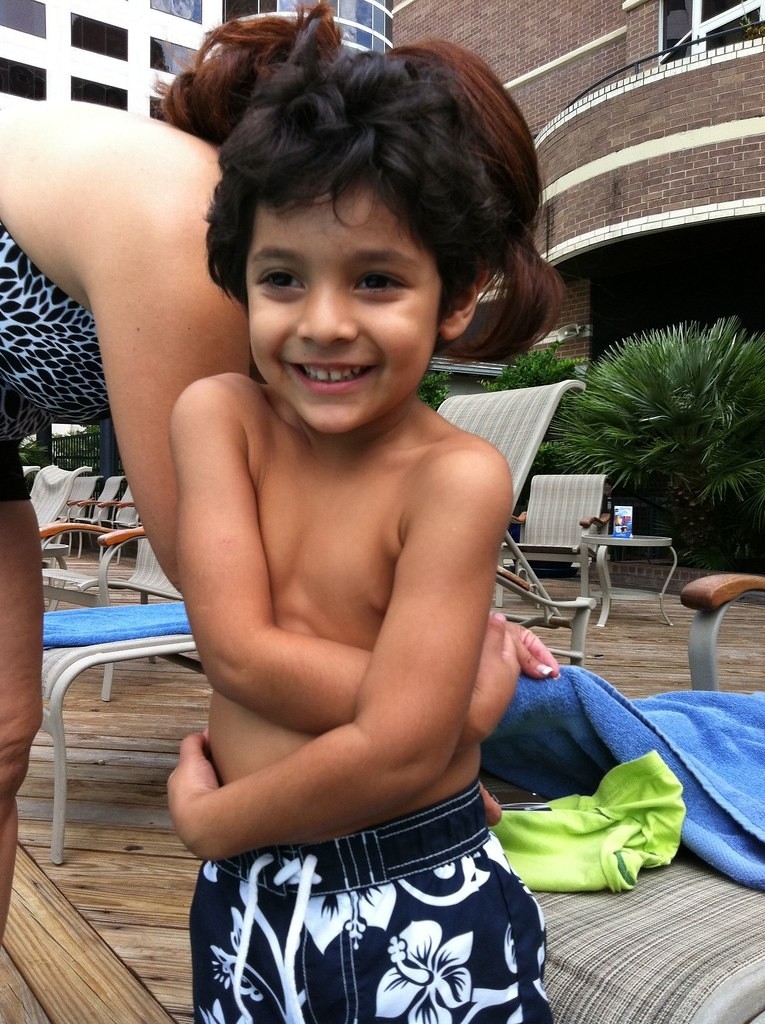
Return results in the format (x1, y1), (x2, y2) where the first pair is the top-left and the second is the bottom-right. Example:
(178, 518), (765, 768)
(0, 1), (561, 949)
(168, 38), (553, 1024)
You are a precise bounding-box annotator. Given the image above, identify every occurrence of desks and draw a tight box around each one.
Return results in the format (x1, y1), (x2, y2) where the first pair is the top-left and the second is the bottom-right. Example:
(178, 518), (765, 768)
(581, 535), (678, 625)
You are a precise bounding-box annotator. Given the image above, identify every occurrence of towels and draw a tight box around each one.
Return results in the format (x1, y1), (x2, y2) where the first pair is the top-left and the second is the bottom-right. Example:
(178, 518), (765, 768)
(40, 601), (194, 651)
(479, 661), (764, 896)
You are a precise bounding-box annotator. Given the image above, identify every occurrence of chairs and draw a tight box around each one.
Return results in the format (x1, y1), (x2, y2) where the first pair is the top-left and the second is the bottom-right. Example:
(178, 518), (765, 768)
(526, 574), (764, 1024)
(21, 381), (608, 868)
